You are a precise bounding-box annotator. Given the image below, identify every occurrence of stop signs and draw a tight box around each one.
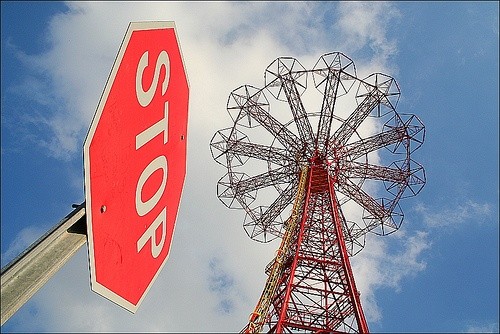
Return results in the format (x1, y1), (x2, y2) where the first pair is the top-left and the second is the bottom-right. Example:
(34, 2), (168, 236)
(82, 19), (191, 314)
